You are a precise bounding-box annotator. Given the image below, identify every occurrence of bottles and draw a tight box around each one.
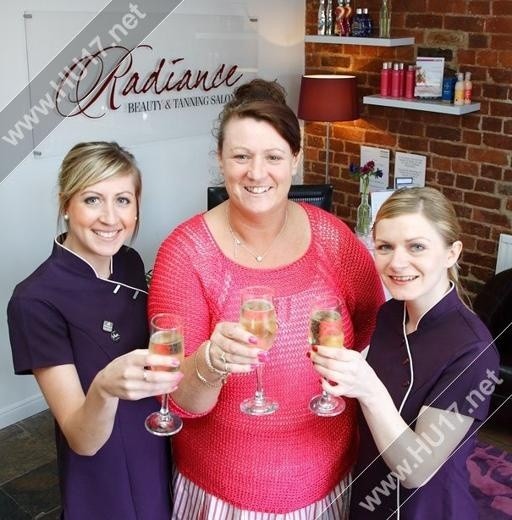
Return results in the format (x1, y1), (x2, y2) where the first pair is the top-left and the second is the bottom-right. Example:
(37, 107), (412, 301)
(443, 72), (473, 106)
(318, 1), (393, 40)
(356, 194), (372, 238)
(381, 62), (416, 100)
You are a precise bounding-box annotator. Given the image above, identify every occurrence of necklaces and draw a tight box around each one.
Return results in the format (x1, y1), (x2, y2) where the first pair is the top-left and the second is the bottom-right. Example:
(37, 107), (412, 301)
(224, 206), (288, 261)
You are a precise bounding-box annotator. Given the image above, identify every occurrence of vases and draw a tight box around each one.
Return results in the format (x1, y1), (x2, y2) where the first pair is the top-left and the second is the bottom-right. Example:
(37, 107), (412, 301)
(355, 193), (373, 236)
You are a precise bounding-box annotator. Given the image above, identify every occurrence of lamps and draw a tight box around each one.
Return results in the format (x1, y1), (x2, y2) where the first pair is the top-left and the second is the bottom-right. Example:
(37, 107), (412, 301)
(298, 74), (359, 187)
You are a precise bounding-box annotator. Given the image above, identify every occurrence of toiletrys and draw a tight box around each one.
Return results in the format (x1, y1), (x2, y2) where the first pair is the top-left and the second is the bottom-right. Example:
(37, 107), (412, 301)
(380, 61), (415, 99)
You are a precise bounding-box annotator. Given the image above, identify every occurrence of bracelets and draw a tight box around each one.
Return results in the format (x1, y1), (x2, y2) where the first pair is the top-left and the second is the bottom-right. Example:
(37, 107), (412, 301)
(194, 340), (230, 387)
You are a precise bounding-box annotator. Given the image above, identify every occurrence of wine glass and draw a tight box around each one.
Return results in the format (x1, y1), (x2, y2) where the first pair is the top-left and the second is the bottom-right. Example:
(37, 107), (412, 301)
(239, 286), (281, 417)
(144, 314), (184, 438)
(306, 297), (346, 417)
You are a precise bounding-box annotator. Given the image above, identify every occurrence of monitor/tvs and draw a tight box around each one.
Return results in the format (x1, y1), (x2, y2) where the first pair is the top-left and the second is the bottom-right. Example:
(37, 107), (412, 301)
(206, 183), (334, 213)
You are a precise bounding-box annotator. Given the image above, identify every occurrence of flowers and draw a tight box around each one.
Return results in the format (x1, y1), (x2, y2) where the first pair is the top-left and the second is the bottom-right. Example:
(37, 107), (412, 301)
(349, 160), (382, 231)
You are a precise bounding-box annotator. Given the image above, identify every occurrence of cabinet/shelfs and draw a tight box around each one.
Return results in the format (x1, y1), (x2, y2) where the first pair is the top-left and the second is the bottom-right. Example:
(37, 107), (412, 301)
(299, 30), (480, 115)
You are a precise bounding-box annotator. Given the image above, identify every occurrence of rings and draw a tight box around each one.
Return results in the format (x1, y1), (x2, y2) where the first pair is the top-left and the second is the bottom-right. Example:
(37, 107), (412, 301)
(143, 371), (148, 383)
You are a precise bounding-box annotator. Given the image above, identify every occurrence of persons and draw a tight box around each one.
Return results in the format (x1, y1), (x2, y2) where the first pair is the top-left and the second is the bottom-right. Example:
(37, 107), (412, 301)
(305, 186), (501, 519)
(7, 141), (182, 520)
(147, 79), (385, 520)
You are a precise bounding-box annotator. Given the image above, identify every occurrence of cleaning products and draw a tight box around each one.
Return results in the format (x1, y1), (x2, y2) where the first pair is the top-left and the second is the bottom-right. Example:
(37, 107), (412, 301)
(441, 71), (473, 105)
(381, 62), (416, 99)
(318, 0), (392, 38)
